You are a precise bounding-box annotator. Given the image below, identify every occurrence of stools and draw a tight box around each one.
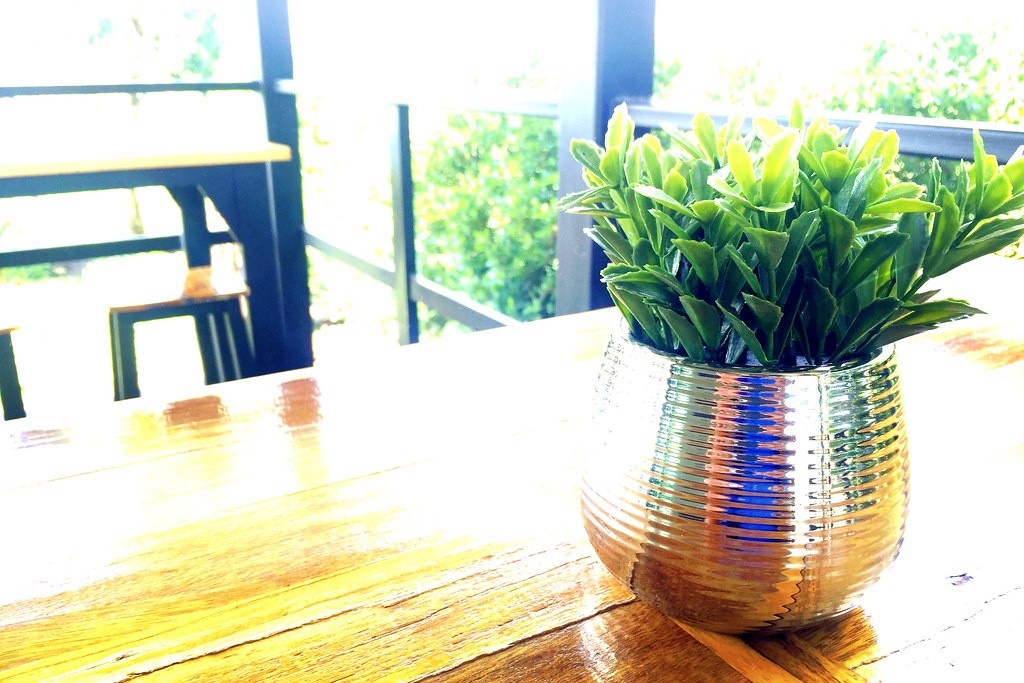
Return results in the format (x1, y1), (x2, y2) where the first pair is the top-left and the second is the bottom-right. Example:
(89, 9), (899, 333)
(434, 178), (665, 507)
(0, 322), (27, 421)
(109, 264), (254, 403)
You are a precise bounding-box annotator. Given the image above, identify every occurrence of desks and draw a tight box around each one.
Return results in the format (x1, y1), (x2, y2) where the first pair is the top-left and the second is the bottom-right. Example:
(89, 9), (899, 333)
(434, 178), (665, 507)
(0, 205), (1024, 682)
(0, 132), (291, 376)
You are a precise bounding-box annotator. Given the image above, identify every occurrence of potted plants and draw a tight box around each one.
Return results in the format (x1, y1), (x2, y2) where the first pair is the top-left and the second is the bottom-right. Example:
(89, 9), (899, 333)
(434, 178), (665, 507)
(557, 100), (1024, 638)
